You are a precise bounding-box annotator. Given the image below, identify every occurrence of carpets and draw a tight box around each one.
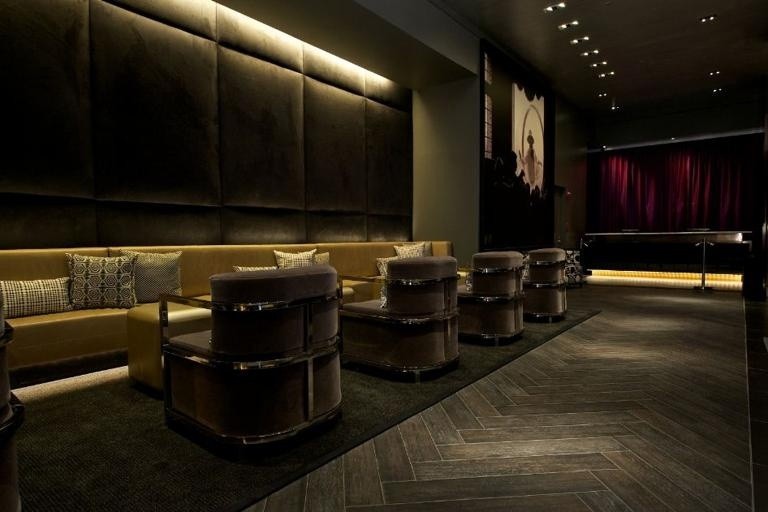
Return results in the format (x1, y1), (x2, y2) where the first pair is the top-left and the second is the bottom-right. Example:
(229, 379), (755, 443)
(16, 306), (603, 512)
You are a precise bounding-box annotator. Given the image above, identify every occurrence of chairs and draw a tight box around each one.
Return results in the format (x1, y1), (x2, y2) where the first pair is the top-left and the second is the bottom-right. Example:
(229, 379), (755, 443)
(523, 248), (569, 322)
(454, 251), (527, 346)
(340, 256), (460, 383)
(159, 269), (342, 455)
